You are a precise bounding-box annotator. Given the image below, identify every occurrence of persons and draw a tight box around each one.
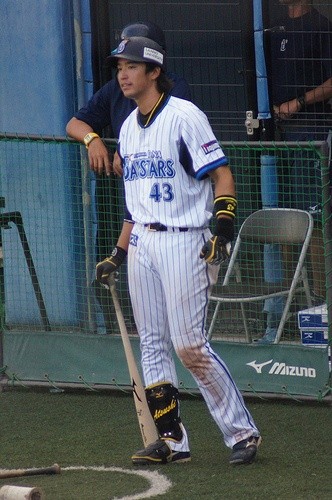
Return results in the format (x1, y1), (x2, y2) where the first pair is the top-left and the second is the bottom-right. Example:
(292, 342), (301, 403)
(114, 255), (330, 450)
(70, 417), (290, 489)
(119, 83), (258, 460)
(67, 23), (193, 335)
(95, 36), (261, 466)
(254, 1), (331, 314)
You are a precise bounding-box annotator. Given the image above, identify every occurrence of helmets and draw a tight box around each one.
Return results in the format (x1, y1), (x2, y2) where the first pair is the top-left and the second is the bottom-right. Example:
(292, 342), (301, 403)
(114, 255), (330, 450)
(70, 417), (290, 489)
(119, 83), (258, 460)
(105, 36), (167, 74)
(119, 19), (166, 50)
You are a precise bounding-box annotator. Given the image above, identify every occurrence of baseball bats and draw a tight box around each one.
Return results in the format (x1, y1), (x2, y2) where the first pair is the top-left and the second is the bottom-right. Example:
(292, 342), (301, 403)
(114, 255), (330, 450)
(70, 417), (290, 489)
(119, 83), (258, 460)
(106, 271), (159, 448)
(0, 463), (61, 479)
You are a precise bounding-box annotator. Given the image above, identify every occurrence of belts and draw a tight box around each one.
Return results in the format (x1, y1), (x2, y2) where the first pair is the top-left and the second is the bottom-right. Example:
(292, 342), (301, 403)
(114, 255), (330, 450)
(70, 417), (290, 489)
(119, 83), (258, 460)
(144, 222), (189, 232)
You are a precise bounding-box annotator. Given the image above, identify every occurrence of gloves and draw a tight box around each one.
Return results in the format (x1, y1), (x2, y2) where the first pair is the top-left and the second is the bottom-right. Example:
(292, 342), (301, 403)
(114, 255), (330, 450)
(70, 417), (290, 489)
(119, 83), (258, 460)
(95, 255), (122, 291)
(200, 234), (232, 266)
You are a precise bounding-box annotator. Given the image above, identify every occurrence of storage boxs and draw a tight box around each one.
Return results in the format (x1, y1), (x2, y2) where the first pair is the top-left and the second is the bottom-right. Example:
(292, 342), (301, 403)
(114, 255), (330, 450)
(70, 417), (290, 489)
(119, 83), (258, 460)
(297, 303), (329, 345)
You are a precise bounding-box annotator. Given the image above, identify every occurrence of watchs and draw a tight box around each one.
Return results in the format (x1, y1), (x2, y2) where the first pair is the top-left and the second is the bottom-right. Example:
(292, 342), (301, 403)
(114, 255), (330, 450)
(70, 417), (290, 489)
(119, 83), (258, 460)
(296, 90), (306, 108)
(84, 132), (98, 146)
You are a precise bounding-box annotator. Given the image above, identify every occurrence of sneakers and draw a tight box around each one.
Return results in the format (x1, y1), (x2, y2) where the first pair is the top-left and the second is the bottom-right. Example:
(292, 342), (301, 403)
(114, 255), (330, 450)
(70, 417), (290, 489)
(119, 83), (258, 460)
(130, 440), (191, 466)
(228, 435), (263, 465)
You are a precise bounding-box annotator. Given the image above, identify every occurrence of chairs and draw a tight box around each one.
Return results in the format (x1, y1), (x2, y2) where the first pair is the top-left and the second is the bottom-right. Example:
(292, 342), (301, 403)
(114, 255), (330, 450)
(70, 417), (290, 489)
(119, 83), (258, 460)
(206, 208), (314, 348)
